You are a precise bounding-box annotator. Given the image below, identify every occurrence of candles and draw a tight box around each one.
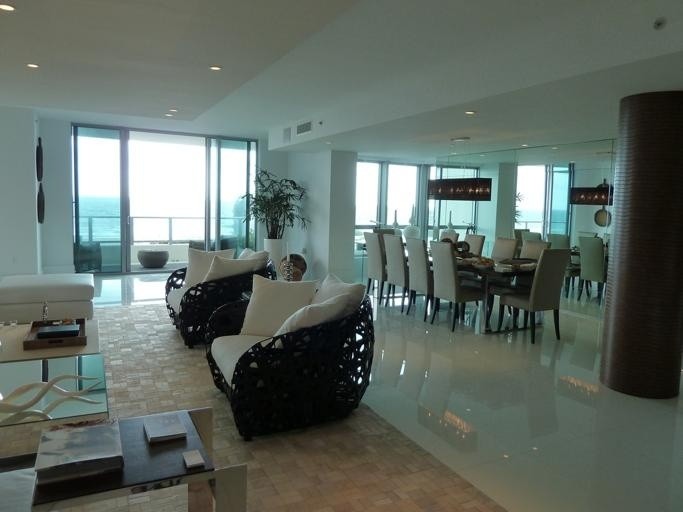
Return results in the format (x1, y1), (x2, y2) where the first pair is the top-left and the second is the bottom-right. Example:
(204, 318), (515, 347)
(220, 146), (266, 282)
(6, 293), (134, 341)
(285, 240), (291, 262)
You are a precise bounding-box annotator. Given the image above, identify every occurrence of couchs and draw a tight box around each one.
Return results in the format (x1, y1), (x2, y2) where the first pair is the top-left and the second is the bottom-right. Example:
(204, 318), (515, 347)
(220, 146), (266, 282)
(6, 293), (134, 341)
(206, 293), (374, 441)
(165, 259), (276, 349)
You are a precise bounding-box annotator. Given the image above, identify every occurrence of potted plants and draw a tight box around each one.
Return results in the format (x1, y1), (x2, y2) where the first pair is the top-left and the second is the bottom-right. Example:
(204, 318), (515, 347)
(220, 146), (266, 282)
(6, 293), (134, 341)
(240, 167), (313, 272)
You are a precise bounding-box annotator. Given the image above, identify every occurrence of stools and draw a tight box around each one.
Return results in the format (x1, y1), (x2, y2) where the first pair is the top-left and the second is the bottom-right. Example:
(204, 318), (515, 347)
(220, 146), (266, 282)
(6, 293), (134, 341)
(0, 273), (94, 325)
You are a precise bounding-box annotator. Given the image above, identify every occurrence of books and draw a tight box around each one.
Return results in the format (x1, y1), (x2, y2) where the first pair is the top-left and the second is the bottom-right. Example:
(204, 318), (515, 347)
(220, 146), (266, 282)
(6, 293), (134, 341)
(180, 448), (205, 468)
(141, 411), (187, 443)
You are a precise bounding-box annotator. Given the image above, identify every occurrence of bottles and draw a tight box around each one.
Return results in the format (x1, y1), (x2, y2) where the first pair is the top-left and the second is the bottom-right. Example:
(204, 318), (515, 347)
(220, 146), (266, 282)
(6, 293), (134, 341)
(265, 260), (275, 279)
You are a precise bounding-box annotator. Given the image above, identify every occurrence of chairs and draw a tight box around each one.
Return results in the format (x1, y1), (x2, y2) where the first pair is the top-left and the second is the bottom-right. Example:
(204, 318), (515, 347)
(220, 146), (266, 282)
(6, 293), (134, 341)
(363, 228), (609, 345)
(369, 308), (603, 439)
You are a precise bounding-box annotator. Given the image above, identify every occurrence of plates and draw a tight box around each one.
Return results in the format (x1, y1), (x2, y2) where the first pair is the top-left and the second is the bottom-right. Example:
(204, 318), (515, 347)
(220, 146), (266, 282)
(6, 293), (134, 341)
(472, 263), (489, 270)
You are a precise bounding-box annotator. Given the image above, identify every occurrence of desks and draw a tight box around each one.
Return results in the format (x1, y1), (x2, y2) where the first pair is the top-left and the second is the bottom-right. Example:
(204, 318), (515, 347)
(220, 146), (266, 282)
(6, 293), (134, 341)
(30, 407), (247, 512)
(136, 250), (169, 268)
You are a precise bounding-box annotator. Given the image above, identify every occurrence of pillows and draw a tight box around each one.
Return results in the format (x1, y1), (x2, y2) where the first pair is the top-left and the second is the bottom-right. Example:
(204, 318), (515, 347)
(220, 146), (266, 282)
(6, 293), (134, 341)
(183, 248), (270, 293)
(238, 273), (366, 337)
